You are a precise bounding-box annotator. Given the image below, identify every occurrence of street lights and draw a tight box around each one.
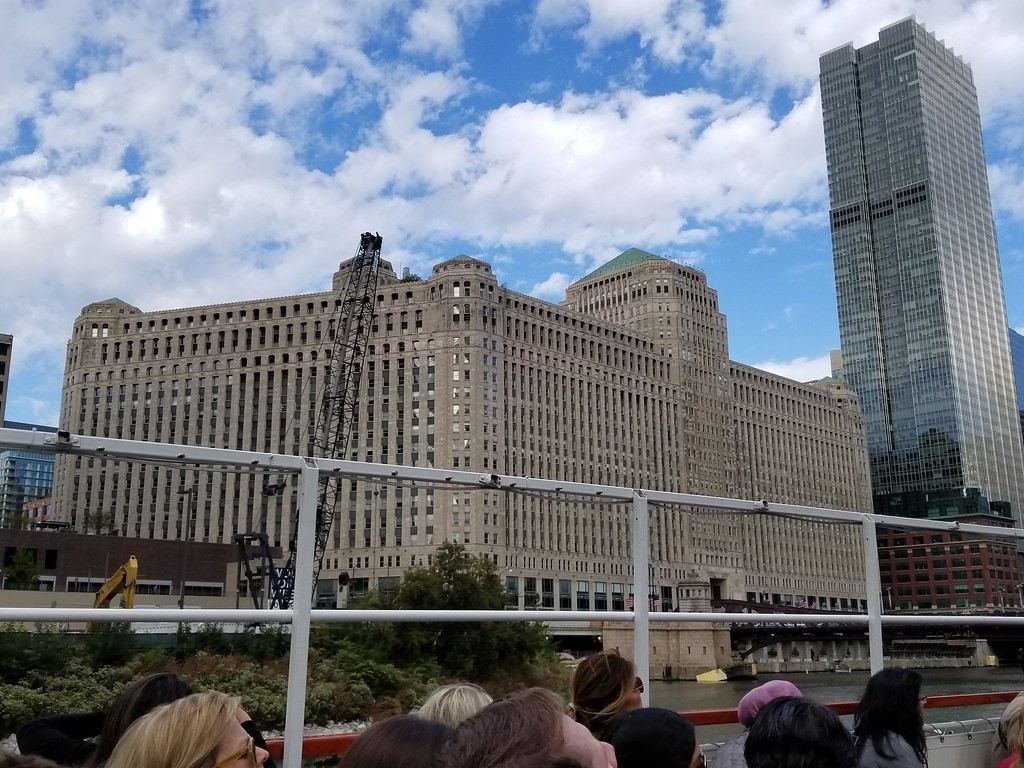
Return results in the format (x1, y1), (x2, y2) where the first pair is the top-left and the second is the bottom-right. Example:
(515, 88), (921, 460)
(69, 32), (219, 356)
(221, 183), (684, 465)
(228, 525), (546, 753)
(648, 564), (655, 612)
(1015, 584), (1023, 611)
(176, 488), (192, 646)
(886, 587), (892, 611)
(2, 577), (7, 591)
(497, 567), (512, 594)
(998, 589), (1007, 614)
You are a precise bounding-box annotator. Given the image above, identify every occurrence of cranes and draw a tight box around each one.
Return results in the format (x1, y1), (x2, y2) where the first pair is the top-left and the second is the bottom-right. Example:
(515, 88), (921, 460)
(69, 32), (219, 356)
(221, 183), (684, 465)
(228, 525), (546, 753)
(229, 231), (383, 609)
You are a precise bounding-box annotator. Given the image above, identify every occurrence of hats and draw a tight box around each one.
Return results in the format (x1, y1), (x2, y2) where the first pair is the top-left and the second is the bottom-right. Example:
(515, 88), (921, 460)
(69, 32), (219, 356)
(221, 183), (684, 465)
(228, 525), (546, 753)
(738, 680), (806, 725)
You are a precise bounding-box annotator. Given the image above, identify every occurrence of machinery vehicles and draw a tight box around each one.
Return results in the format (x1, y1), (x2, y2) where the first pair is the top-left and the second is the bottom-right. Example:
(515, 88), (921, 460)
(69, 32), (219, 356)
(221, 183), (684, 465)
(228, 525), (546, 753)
(83, 555), (138, 633)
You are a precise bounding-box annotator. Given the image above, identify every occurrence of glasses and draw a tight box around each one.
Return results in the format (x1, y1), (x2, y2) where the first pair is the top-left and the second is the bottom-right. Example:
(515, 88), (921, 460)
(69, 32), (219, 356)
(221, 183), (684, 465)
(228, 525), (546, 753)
(631, 676), (645, 693)
(212, 737), (258, 768)
(917, 695), (928, 703)
(699, 748), (708, 768)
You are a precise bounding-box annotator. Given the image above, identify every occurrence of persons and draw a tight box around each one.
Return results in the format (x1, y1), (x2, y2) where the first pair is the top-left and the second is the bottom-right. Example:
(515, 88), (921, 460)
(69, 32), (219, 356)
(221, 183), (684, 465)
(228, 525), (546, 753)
(568, 646), (643, 741)
(709, 678), (805, 768)
(992, 690), (1024, 768)
(744, 696), (857, 768)
(847, 665), (929, 768)
(1, 672), (280, 768)
(337, 714), (455, 768)
(436, 685), (618, 768)
(600, 705), (710, 768)
(411, 682), (494, 730)
